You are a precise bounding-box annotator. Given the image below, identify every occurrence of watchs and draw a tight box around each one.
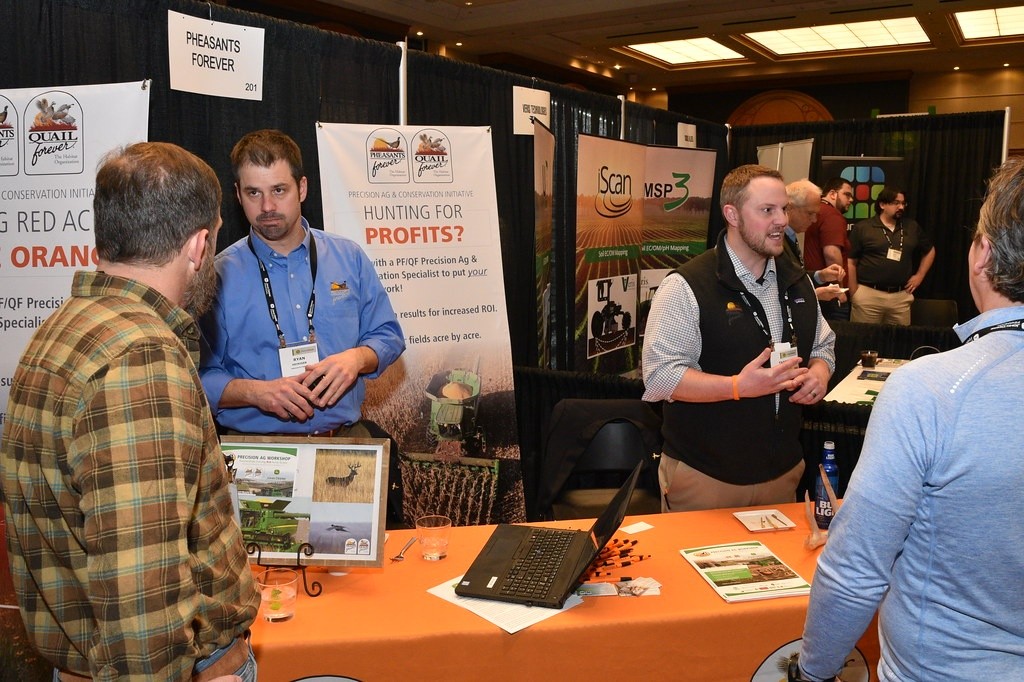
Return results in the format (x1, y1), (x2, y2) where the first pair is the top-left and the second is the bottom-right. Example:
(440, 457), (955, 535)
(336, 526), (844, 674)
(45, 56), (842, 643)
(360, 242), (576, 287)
(788, 663), (836, 682)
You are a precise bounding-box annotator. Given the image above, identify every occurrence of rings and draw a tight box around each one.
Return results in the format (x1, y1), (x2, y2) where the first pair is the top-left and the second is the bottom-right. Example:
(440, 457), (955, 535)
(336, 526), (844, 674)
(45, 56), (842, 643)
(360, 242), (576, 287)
(810, 391), (817, 397)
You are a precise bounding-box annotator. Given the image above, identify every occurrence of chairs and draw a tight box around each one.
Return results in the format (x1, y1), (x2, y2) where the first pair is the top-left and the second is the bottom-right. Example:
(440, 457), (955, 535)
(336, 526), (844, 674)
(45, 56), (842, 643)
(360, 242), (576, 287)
(912, 299), (959, 328)
(538, 400), (665, 521)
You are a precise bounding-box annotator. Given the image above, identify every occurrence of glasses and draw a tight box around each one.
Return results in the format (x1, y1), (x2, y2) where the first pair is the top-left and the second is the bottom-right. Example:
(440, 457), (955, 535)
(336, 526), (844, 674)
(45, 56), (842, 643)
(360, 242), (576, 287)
(835, 190), (853, 199)
(889, 201), (908, 207)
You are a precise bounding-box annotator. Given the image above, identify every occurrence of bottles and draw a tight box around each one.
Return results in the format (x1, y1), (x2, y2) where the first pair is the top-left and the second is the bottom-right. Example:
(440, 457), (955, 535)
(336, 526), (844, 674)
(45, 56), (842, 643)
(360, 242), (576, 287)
(815, 442), (839, 529)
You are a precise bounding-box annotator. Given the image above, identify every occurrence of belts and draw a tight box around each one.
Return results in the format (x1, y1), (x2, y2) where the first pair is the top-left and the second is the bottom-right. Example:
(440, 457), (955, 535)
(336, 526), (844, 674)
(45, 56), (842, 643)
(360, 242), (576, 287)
(285, 431), (337, 438)
(59, 631), (248, 682)
(857, 280), (907, 293)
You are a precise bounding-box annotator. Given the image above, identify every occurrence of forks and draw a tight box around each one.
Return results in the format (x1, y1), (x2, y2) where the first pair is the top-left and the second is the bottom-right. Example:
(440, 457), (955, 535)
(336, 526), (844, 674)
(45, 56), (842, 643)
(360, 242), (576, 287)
(389, 537), (416, 561)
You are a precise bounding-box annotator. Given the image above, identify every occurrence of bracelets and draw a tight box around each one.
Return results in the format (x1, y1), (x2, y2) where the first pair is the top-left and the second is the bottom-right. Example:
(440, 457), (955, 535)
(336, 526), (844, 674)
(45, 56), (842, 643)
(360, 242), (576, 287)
(733, 376), (739, 400)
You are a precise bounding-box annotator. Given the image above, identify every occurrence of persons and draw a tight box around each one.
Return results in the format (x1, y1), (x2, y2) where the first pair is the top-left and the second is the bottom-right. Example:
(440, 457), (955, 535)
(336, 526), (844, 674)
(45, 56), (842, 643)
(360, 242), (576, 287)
(200, 130), (407, 436)
(849, 187), (936, 326)
(0, 143), (263, 682)
(783, 180), (846, 302)
(798, 156), (1024, 682)
(804, 178), (853, 320)
(642, 165), (836, 511)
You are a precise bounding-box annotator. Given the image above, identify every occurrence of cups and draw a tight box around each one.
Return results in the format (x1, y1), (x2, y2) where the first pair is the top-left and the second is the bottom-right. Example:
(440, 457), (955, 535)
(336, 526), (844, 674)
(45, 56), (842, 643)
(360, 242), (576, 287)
(416, 516), (452, 560)
(861, 351), (877, 370)
(256, 569), (299, 623)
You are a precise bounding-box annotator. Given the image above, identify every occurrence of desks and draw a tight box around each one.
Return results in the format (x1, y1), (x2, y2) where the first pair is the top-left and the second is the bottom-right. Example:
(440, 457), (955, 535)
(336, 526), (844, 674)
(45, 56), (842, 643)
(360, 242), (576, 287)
(823, 358), (913, 407)
(250, 499), (883, 682)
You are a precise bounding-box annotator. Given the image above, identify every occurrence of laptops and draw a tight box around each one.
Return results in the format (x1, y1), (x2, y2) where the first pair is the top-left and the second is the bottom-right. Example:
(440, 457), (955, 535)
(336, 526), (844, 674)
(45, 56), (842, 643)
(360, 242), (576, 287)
(455, 459), (644, 609)
